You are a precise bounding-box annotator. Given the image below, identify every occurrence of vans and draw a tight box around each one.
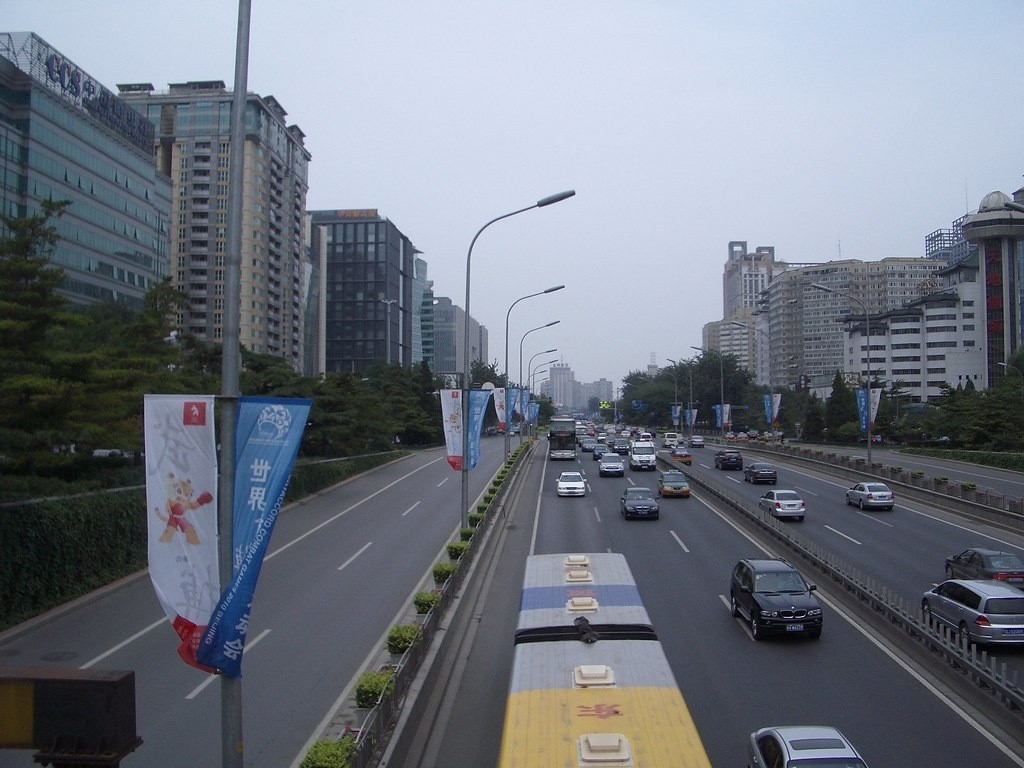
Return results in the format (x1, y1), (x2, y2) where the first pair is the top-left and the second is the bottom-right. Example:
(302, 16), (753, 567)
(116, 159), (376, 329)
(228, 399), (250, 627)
(660, 433), (679, 447)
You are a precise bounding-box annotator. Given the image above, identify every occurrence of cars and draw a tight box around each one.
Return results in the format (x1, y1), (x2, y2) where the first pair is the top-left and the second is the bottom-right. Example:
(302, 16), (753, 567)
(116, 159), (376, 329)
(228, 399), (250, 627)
(921, 577), (1024, 651)
(574, 415), (656, 461)
(747, 726), (870, 768)
(555, 472), (587, 497)
(860, 435), (885, 446)
(598, 452), (625, 477)
(687, 436), (705, 448)
(677, 434), (684, 444)
(758, 490), (806, 521)
(657, 469), (690, 498)
(743, 463), (778, 485)
(619, 487), (660, 520)
(845, 481), (895, 511)
(488, 425), (503, 437)
(724, 430), (781, 443)
(946, 547), (1024, 589)
(669, 448), (691, 465)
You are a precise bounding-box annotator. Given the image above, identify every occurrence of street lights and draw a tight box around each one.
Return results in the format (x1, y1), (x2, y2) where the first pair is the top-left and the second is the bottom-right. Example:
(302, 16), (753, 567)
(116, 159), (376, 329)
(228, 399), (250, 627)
(520, 320), (559, 447)
(461, 189), (577, 538)
(503, 285), (564, 468)
(625, 321), (775, 451)
(811, 283), (872, 474)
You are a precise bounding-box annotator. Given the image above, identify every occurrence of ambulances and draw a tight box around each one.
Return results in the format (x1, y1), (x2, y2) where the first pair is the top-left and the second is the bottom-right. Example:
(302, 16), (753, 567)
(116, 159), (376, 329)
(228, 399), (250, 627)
(628, 439), (657, 471)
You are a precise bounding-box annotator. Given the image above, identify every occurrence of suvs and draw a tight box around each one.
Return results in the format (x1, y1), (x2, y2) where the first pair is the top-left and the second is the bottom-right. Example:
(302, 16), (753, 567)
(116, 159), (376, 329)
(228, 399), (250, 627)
(730, 556), (823, 641)
(713, 450), (743, 470)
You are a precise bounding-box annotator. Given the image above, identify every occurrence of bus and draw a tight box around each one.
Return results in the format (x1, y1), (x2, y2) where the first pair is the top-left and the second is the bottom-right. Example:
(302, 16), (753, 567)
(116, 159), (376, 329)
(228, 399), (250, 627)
(498, 551), (713, 768)
